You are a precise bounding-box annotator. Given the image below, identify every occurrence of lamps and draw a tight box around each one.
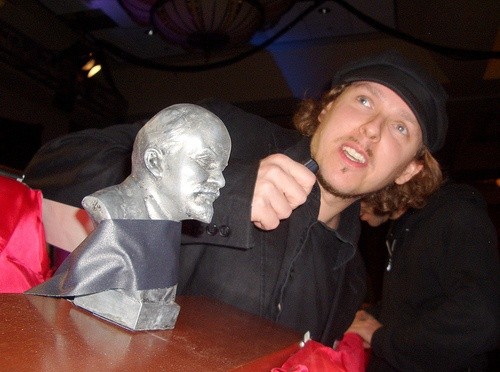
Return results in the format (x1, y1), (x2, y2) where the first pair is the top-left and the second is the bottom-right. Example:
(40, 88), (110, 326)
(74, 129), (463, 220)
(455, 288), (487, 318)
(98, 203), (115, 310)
(117, 0), (298, 63)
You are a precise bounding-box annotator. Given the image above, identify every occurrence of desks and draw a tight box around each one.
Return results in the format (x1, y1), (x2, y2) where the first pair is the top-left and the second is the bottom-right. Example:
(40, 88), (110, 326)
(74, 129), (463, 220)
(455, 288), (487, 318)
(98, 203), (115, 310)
(0, 292), (310, 372)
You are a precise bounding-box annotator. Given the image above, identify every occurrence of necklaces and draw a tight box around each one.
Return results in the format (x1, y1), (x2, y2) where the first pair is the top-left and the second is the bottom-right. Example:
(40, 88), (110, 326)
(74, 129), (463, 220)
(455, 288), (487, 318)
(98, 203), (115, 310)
(386, 241), (395, 270)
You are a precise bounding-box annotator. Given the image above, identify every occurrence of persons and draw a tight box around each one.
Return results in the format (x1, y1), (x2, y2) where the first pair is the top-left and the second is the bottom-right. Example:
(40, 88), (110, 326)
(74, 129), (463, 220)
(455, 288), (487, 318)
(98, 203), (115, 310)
(23, 54), (449, 349)
(345, 177), (500, 372)
(82, 104), (231, 304)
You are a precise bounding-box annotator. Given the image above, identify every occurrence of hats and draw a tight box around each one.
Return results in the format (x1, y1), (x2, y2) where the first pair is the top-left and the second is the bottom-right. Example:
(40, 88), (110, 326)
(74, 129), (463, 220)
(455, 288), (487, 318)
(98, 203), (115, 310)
(331, 49), (449, 155)
